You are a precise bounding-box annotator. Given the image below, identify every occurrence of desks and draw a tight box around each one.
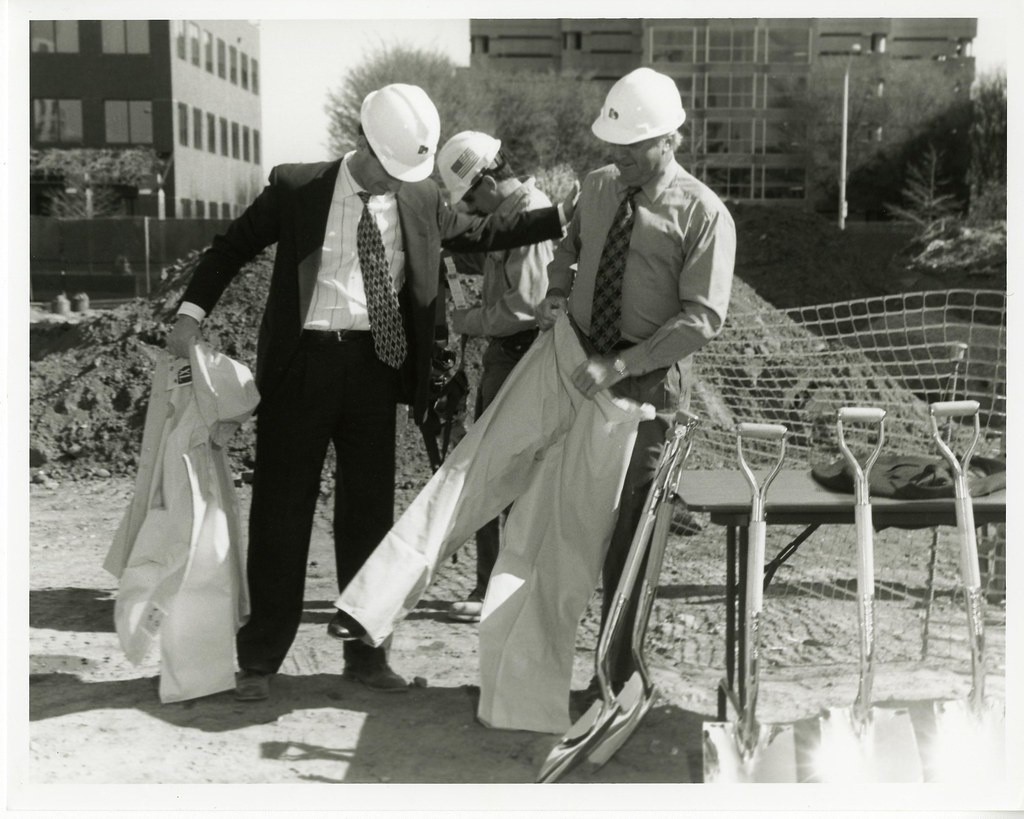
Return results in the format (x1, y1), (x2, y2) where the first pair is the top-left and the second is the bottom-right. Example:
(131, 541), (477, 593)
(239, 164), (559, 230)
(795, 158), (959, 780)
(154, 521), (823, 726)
(676, 467), (1007, 718)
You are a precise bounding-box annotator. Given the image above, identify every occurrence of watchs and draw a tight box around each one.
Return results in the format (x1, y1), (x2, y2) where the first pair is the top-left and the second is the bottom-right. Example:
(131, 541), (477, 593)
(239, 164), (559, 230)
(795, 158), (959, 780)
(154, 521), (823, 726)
(613, 356), (626, 380)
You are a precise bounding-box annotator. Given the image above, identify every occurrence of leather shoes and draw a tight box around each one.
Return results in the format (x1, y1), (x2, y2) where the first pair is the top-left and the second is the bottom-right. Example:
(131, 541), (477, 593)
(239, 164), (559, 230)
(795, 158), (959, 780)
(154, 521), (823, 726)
(327, 609), (366, 641)
(580, 676), (624, 708)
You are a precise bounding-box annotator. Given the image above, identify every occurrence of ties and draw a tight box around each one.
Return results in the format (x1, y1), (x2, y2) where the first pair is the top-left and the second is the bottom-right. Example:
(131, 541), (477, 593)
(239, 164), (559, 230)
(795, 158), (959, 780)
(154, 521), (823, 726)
(589, 185), (642, 358)
(357, 191), (407, 371)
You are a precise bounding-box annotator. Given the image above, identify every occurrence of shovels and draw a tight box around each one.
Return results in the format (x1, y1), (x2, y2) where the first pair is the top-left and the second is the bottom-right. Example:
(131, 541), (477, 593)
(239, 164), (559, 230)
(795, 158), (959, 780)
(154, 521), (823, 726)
(929, 400), (1005, 783)
(582, 416), (700, 777)
(819, 407), (924, 781)
(537, 409), (700, 783)
(702, 423), (798, 784)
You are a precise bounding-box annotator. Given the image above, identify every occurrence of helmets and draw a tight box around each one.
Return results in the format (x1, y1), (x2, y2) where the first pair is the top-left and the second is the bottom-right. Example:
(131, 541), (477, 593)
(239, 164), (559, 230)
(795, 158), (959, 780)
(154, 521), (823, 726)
(361, 83), (441, 183)
(592, 68), (686, 145)
(436, 130), (501, 205)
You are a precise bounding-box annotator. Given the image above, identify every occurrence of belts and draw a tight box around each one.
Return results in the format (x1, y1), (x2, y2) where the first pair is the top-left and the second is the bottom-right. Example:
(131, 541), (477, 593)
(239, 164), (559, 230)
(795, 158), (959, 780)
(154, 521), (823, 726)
(587, 337), (637, 352)
(301, 330), (371, 343)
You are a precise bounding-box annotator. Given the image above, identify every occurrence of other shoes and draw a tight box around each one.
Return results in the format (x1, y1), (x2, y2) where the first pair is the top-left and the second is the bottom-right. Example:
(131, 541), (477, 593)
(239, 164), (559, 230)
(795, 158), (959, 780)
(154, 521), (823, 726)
(235, 671), (277, 700)
(343, 664), (407, 693)
(447, 587), (485, 621)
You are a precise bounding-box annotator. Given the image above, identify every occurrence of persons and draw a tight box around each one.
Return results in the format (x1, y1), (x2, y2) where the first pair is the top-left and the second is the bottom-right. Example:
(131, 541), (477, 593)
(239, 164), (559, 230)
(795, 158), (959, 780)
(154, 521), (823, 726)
(167, 83), (583, 697)
(437, 131), (556, 623)
(327, 67), (738, 714)
